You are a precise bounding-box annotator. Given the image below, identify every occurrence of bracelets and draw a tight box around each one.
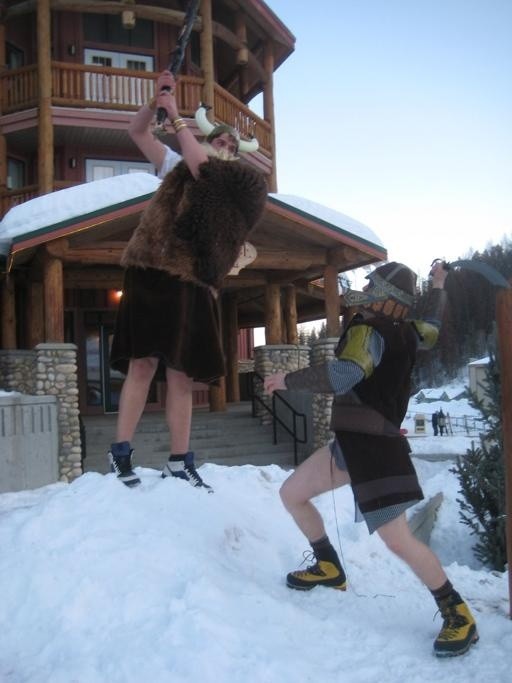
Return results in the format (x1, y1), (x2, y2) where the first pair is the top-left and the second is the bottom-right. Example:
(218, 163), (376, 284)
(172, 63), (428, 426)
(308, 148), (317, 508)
(149, 96), (158, 110)
(171, 117), (187, 132)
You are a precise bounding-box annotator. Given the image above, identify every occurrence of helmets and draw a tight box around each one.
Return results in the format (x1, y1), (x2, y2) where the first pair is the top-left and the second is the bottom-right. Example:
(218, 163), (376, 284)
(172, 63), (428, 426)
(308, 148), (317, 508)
(362, 262), (417, 321)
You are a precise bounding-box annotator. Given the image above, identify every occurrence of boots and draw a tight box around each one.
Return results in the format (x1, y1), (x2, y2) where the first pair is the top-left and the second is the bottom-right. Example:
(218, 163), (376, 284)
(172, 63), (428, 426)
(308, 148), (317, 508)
(287, 545), (346, 591)
(433, 593), (479, 657)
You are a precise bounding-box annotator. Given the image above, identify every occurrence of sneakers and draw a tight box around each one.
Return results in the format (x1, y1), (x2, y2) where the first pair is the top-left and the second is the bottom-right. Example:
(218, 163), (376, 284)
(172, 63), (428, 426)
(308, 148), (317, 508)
(108, 441), (141, 488)
(162, 452), (213, 493)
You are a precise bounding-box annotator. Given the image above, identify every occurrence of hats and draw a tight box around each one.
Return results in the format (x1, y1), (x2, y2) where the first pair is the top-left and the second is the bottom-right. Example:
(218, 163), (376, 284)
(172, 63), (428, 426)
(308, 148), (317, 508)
(207, 126), (240, 155)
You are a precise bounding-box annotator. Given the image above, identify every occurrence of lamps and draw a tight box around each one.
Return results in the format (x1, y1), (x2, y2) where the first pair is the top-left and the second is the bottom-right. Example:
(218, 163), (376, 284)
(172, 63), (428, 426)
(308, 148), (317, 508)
(235, 47), (249, 66)
(120, 10), (135, 27)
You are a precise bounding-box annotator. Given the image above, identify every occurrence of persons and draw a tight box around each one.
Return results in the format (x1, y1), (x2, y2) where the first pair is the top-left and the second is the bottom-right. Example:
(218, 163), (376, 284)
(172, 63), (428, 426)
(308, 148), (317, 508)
(107, 69), (269, 493)
(437, 409), (448, 436)
(279, 262), (479, 657)
(432, 410), (440, 436)
